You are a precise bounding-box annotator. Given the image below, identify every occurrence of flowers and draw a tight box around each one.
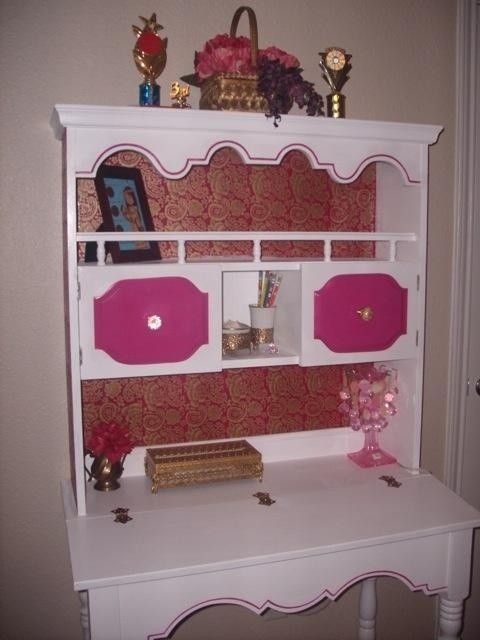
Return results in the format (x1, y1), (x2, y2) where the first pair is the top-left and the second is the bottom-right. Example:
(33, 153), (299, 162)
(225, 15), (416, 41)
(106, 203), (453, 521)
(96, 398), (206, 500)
(88, 421), (135, 464)
(193, 33), (325, 127)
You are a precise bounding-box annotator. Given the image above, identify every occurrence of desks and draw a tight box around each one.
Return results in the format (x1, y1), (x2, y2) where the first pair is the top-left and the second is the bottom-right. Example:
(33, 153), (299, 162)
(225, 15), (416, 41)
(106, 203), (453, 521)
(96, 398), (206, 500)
(61, 472), (479, 640)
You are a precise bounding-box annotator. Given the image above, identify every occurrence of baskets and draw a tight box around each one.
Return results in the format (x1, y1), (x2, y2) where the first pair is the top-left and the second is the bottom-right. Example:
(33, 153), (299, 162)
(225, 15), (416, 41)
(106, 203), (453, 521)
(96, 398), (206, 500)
(199, 7), (293, 113)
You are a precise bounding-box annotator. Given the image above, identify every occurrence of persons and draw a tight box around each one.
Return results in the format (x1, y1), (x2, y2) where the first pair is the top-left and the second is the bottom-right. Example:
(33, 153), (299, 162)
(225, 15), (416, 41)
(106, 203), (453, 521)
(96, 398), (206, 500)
(117, 186), (146, 250)
(119, 205), (137, 222)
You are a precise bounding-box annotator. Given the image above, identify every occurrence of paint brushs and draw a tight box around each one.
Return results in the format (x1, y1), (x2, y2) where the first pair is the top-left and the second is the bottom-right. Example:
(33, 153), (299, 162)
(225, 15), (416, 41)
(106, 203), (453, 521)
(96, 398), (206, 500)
(258, 270), (282, 308)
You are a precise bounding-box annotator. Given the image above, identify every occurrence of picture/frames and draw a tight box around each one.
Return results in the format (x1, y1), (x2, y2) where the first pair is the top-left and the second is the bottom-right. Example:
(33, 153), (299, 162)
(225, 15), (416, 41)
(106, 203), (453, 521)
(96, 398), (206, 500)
(93, 166), (160, 264)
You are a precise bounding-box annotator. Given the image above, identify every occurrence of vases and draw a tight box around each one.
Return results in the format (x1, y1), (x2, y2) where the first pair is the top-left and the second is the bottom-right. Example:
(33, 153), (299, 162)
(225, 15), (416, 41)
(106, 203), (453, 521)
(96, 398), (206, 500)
(91, 452), (125, 491)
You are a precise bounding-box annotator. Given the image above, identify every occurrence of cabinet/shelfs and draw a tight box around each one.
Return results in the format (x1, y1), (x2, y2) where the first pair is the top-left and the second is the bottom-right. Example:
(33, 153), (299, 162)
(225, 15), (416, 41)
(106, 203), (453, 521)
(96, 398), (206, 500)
(66, 127), (429, 515)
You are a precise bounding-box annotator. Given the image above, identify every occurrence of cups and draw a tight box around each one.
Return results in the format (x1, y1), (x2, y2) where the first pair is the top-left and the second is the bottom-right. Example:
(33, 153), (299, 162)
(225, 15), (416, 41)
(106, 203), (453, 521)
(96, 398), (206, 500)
(222, 323), (251, 357)
(249, 304), (277, 350)
